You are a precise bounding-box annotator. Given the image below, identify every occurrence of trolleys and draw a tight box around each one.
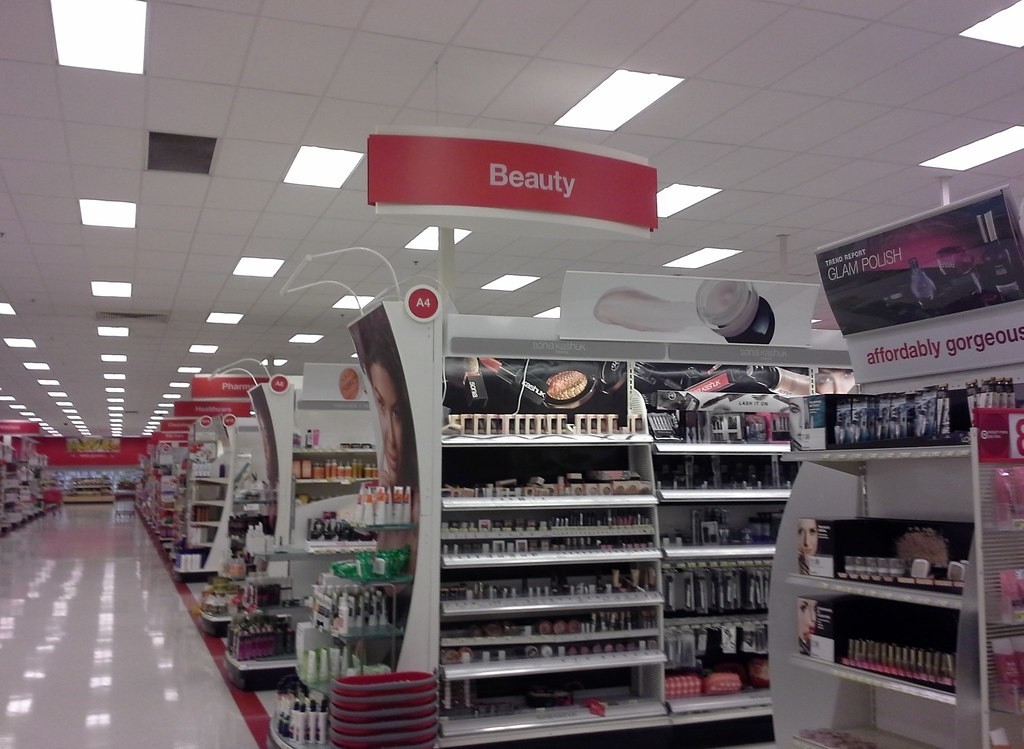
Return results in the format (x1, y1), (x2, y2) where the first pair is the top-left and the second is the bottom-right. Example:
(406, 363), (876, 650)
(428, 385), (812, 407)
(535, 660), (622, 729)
(42, 486), (63, 517)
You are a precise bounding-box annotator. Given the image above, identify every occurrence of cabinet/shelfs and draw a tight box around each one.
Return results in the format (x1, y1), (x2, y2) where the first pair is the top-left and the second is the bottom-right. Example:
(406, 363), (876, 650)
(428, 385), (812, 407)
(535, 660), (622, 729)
(0, 459), (62, 539)
(134, 297), (797, 739)
(779, 438), (1024, 745)
(69, 476), (112, 495)
